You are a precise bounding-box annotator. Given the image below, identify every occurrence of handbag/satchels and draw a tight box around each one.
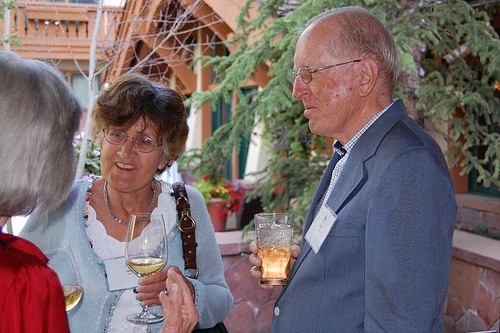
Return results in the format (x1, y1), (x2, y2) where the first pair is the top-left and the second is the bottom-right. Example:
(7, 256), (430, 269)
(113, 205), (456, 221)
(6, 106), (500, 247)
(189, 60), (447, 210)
(171, 182), (227, 333)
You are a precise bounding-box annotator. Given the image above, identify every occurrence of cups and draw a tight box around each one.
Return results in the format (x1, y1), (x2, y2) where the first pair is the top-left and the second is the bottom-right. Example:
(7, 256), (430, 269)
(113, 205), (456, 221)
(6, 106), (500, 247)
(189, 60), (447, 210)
(254, 213), (294, 286)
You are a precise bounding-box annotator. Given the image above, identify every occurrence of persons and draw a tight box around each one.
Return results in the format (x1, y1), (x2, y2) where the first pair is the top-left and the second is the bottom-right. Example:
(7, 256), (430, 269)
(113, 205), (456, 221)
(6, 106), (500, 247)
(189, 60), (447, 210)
(0, 50), (83, 333)
(17, 73), (233, 333)
(249, 6), (457, 333)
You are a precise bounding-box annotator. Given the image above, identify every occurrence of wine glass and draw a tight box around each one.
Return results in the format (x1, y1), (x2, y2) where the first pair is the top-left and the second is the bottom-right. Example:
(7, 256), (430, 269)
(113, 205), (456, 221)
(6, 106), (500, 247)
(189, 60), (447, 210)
(35, 241), (84, 314)
(124, 213), (168, 324)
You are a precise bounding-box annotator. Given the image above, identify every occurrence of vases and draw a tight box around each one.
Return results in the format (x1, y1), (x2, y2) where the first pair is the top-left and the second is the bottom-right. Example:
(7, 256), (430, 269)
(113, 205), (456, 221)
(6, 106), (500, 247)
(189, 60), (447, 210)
(206, 198), (227, 232)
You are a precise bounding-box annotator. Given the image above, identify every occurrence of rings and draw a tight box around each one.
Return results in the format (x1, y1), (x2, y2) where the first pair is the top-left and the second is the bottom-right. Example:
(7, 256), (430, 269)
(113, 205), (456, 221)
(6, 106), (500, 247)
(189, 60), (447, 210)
(164, 287), (168, 296)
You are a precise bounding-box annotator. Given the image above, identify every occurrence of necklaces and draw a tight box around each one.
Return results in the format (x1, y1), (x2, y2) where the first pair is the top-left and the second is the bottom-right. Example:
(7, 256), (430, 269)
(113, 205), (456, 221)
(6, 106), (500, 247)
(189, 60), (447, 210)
(104, 177), (156, 227)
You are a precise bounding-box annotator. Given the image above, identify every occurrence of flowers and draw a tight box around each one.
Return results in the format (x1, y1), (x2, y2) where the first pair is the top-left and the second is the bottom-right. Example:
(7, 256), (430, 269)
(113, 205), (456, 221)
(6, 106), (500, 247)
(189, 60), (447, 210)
(186, 168), (245, 220)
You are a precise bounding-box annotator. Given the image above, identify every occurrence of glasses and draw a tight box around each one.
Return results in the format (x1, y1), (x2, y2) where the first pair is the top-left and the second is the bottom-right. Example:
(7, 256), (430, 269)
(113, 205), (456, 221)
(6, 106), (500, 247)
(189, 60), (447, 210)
(102, 127), (163, 153)
(291, 60), (362, 83)
